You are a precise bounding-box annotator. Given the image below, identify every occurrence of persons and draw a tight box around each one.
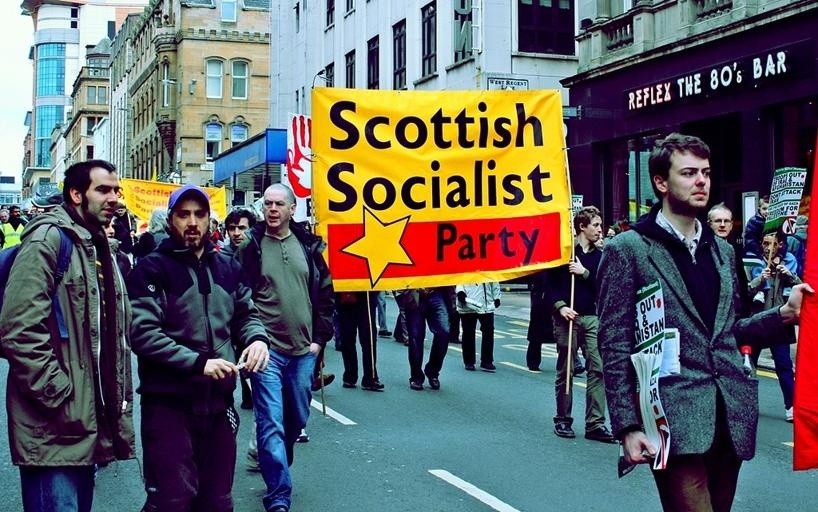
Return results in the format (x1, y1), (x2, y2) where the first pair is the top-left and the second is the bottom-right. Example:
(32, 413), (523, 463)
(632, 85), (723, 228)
(1, 160), (501, 511)
(528, 134), (816, 511)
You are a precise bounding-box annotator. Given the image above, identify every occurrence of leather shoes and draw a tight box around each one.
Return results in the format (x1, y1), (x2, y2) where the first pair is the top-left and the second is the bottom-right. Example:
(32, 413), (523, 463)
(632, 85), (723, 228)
(574, 366), (585, 375)
(312, 374), (335, 391)
(379, 330), (392, 335)
(410, 381), (423, 390)
(555, 423), (575, 437)
(585, 426), (614, 443)
(430, 377), (440, 389)
(361, 381), (384, 389)
(403, 339), (409, 344)
(343, 382), (355, 388)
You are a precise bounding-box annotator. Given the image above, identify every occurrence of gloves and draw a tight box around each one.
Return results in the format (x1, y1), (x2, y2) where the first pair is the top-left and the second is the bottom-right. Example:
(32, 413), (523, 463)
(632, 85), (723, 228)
(495, 299), (500, 308)
(457, 291), (467, 306)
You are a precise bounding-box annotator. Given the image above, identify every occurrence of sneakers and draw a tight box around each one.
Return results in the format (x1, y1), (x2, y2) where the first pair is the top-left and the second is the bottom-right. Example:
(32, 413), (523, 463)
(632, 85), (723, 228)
(785, 406), (796, 421)
(246, 448), (261, 473)
(480, 364), (497, 372)
(465, 363), (474, 371)
(296, 428), (310, 442)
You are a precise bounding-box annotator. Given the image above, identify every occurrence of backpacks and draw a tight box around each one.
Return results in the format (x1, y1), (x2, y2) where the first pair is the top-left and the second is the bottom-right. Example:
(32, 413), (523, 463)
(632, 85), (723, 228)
(0, 224), (75, 340)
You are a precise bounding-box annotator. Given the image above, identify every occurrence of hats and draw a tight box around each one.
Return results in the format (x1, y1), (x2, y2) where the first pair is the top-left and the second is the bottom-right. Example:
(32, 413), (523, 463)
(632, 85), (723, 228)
(168, 185), (209, 211)
(31, 190), (63, 208)
